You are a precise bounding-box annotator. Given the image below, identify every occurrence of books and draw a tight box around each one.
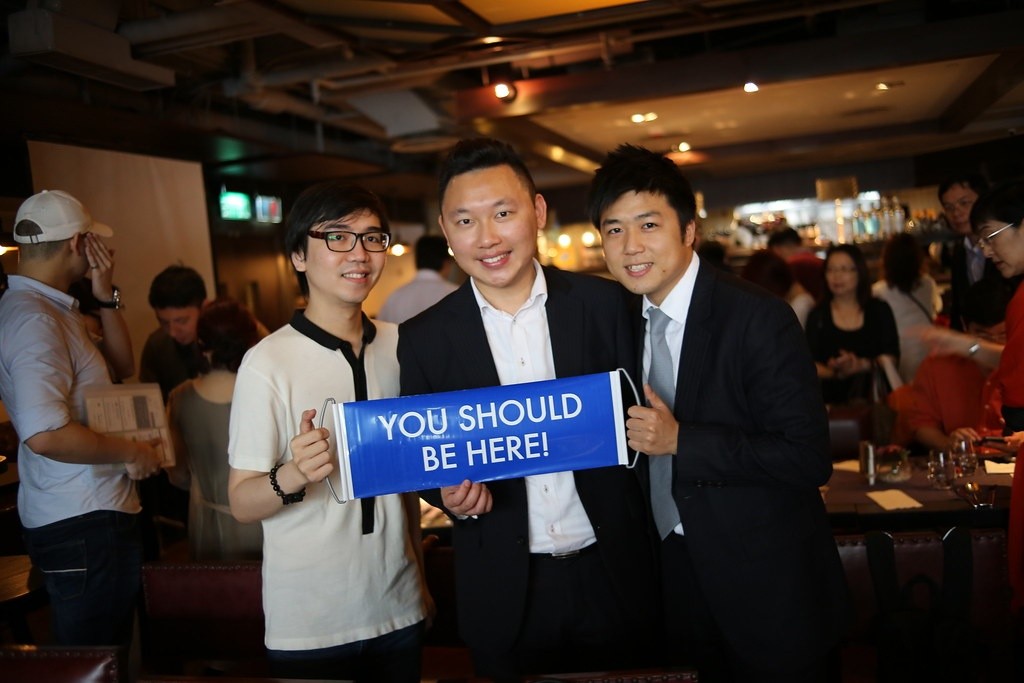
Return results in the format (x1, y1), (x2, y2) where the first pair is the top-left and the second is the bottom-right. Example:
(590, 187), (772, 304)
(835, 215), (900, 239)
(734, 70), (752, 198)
(82, 383), (176, 478)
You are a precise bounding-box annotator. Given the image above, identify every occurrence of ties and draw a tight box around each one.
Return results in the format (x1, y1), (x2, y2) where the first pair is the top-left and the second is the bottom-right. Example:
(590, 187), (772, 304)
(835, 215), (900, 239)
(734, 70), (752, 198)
(646, 305), (680, 542)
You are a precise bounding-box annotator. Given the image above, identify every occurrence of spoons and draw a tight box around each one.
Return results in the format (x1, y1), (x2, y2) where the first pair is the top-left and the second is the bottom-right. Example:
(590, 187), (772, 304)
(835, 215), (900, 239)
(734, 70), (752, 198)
(955, 481), (987, 507)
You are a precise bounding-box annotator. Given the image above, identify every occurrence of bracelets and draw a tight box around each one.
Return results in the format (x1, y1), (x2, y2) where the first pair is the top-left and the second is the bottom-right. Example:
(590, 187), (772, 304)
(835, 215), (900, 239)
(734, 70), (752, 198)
(269, 463), (306, 505)
(967, 338), (983, 361)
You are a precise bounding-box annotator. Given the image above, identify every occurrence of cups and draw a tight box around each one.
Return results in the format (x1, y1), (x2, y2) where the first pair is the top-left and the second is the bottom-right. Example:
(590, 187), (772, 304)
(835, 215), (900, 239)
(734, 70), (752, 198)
(919, 435), (978, 490)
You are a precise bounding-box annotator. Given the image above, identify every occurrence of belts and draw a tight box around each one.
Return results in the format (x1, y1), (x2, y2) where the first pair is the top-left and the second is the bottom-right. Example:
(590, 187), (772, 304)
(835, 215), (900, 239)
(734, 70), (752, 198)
(531, 541), (600, 561)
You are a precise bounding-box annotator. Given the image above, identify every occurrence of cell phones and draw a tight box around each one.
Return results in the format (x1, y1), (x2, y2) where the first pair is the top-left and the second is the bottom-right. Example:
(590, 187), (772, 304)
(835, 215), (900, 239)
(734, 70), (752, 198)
(977, 436), (1006, 446)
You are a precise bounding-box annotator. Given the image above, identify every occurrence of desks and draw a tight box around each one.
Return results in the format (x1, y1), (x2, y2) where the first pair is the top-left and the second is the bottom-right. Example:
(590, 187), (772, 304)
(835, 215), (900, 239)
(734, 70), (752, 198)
(819, 458), (1011, 681)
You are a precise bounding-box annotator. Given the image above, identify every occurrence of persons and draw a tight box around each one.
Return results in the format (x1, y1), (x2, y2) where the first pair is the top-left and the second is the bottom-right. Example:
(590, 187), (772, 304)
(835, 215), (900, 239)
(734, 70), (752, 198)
(902, 179), (1023, 633)
(697, 162), (1024, 453)
(377, 232), (461, 325)
(397, 134), (696, 683)
(0, 189), (163, 683)
(590, 142), (855, 683)
(226, 182), (431, 683)
(66, 260), (269, 562)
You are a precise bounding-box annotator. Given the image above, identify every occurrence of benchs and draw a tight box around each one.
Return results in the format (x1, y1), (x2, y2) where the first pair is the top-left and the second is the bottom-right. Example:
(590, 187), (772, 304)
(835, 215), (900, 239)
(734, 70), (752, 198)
(832, 528), (1008, 642)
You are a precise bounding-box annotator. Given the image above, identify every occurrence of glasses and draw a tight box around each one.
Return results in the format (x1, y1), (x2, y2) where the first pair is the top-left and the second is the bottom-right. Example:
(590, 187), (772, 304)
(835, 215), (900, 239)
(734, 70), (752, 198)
(302, 227), (392, 253)
(978, 223), (1014, 248)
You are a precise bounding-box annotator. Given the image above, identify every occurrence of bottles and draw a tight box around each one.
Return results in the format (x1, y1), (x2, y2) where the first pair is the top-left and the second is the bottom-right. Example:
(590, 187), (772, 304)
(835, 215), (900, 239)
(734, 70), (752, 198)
(724, 187), (944, 250)
(858, 441), (875, 478)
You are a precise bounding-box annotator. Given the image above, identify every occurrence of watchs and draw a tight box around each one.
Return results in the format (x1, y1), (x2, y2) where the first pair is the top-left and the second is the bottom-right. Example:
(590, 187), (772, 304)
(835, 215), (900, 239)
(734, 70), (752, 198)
(98, 290), (121, 310)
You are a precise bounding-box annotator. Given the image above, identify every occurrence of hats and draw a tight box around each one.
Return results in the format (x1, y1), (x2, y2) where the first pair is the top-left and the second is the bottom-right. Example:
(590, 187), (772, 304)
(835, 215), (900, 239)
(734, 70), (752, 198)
(14, 188), (114, 244)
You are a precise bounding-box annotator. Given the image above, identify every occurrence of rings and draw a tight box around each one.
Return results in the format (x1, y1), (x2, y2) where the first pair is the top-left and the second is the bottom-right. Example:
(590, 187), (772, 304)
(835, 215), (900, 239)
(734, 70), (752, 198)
(89, 263), (99, 269)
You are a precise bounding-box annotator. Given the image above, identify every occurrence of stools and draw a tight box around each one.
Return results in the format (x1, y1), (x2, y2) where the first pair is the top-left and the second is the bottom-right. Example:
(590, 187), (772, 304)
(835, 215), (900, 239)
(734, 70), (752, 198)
(0, 541), (262, 683)
